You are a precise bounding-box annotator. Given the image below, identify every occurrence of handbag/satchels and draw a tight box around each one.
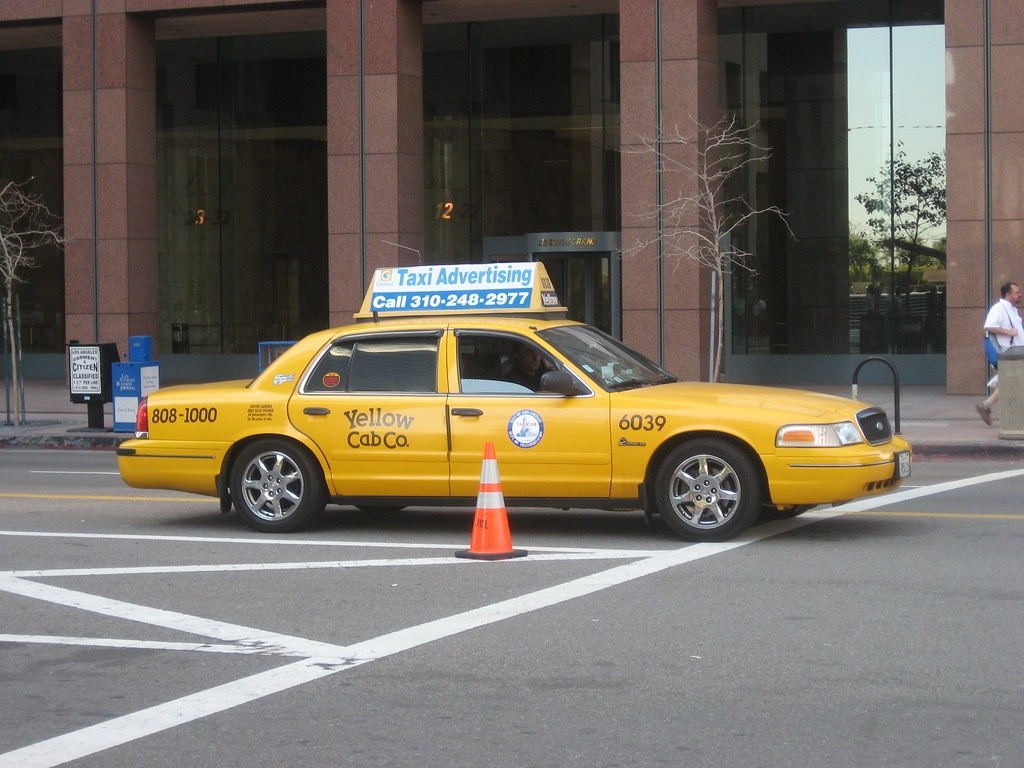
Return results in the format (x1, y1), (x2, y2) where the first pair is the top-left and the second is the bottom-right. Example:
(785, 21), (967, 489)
(984, 331), (1004, 369)
(987, 374), (998, 387)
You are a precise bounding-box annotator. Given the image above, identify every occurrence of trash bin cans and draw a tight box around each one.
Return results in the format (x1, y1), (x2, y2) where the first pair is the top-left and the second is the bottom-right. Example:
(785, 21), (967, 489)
(998, 346), (1024, 441)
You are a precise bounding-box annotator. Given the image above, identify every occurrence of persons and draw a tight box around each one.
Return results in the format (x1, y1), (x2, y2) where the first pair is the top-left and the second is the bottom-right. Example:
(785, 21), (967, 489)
(975, 283), (1024, 426)
(509, 342), (551, 392)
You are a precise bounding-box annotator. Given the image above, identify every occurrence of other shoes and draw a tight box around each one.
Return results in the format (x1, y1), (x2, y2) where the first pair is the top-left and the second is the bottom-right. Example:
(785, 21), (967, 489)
(977, 404), (991, 425)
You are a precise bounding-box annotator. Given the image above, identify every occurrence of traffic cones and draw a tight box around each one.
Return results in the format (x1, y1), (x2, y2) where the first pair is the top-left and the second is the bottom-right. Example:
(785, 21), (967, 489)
(454, 442), (529, 561)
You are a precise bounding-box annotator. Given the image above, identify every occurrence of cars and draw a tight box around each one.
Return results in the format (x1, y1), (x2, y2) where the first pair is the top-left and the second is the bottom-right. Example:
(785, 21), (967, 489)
(117, 262), (914, 543)
